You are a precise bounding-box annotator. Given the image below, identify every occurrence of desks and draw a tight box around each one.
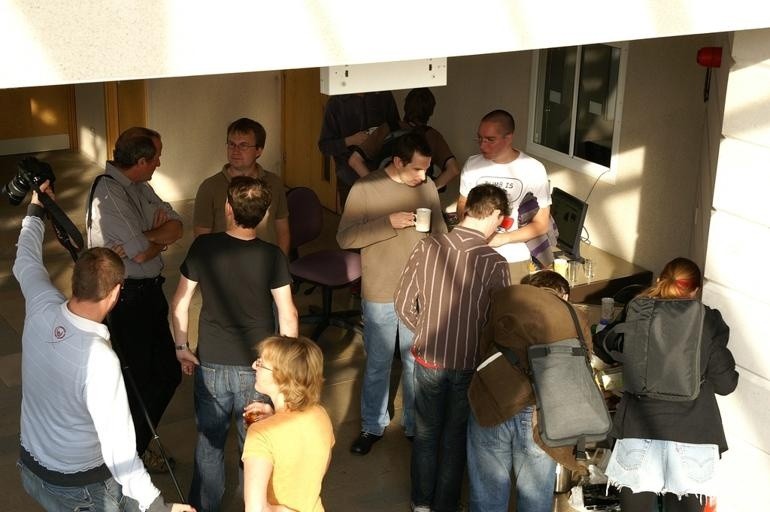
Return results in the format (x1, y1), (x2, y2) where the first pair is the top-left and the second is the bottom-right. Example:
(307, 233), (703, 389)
(488, 240), (653, 305)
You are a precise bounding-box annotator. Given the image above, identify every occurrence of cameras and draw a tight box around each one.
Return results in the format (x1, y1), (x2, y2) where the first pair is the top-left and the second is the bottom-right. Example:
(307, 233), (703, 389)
(2, 156), (56, 206)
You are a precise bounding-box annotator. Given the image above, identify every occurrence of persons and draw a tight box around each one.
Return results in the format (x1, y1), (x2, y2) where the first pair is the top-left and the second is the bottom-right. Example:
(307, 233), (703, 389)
(607, 262), (740, 512)
(193, 118), (292, 266)
(349, 87), (459, 191)
(172, 177), (298, 511)
(335, 135), (450, 454)
(458, 109), (552, 287)
(85, 127), (184, 461)
(13, 181), (197, 512)
(318, 91), (403, 220)
(240, 336), (335, 511)
(393, 184), (513, 512)
(467, 271), (593, 511)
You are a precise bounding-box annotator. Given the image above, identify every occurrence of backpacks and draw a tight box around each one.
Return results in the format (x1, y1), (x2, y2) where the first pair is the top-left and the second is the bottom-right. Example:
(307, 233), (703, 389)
(378, 120), (430, 172)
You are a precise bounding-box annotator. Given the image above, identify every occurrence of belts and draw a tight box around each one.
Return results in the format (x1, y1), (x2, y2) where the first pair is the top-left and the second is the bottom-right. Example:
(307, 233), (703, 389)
(128, 276), (166, 291)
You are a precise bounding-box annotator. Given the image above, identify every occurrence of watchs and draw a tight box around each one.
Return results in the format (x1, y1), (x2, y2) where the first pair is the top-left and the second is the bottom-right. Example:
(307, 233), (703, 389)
(174, 342), (189, 350)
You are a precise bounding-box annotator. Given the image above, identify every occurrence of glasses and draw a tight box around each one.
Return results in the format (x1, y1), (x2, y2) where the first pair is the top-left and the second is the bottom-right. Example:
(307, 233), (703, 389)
(253, 358), (277, 377)
(472, 133), (499, 146)
(224, 140), (259, 151)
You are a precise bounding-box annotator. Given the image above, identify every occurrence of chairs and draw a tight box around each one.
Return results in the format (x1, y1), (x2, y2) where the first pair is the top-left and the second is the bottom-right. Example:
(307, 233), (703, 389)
(286, 188), (363, 346)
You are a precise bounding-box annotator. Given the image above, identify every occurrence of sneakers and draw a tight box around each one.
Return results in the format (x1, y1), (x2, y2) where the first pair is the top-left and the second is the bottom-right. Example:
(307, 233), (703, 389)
(352, 428), (381, 455)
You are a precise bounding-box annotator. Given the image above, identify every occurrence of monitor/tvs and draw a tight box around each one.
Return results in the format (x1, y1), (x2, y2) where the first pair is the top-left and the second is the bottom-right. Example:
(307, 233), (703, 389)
(550, 187), (588, 255)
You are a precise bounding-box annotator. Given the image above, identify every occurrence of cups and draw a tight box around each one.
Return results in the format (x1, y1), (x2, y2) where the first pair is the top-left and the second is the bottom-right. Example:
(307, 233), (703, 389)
(242, 393), (270, 431)
(411, 208), (431, 232)
(584, 257), (597, 282)
(554, 258), (571, 280)
(568, 261), (580, 285)
(601, 298), (615, 319)
